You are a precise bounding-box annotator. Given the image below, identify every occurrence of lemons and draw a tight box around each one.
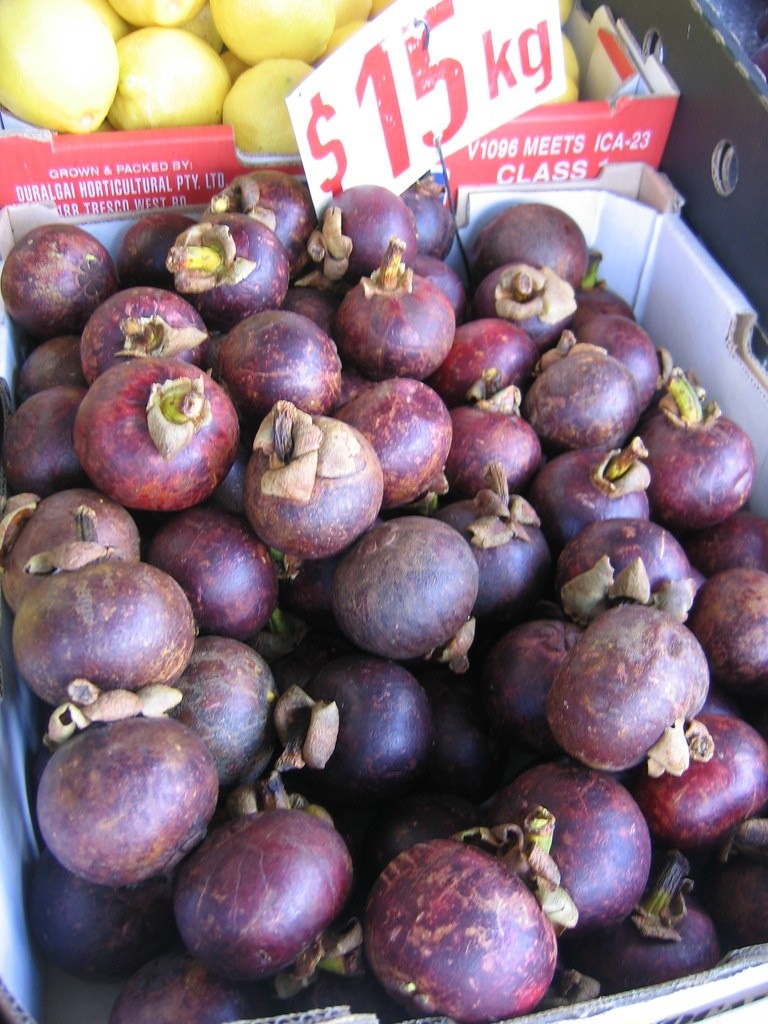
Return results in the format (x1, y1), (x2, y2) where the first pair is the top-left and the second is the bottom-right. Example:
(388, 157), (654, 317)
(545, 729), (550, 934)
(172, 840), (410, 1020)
(0, 0), (581, 155)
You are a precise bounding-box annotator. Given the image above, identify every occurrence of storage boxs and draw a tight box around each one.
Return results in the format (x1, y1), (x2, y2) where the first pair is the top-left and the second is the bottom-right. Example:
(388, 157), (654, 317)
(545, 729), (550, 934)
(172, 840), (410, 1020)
(0, 160), (768, 1024)
(0, 0), (681, 217)
